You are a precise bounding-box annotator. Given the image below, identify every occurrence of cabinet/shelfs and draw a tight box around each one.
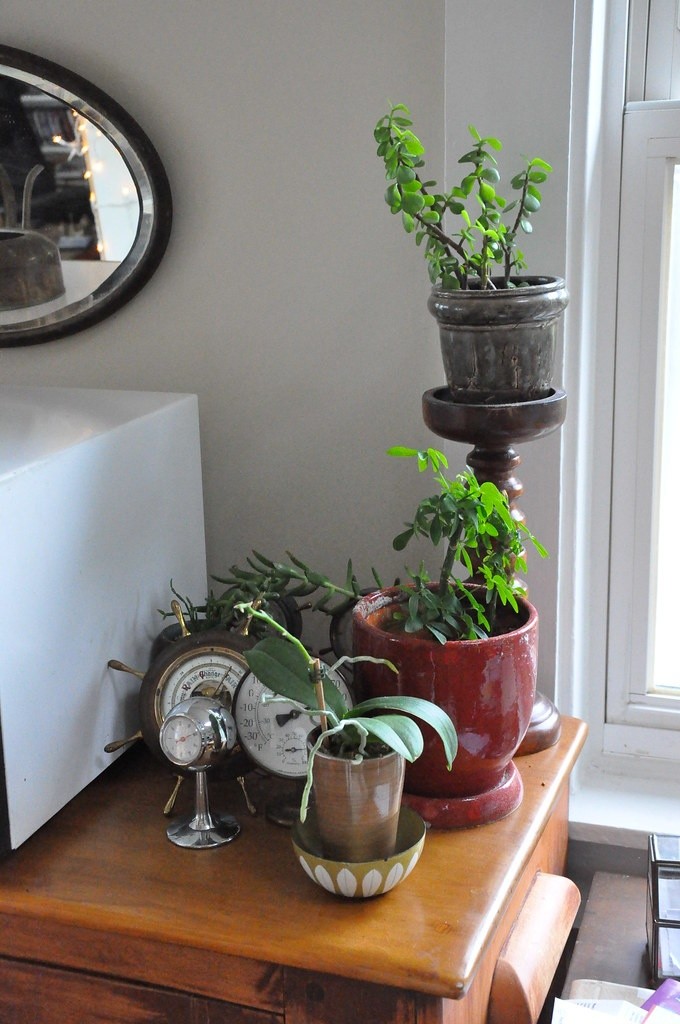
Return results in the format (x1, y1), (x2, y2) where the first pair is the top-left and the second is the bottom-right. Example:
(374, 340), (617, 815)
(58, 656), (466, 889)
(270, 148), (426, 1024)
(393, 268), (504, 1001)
(0, 714), (589, 1024)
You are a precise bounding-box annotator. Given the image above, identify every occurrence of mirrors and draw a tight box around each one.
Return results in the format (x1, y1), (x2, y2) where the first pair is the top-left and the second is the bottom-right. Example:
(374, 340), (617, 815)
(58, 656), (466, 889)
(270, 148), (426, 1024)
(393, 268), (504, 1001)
(0, 46), (174, 348)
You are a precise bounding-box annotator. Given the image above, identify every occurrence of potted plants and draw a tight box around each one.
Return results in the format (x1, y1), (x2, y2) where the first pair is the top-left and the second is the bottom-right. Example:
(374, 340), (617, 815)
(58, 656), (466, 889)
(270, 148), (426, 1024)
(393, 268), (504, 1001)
(228, 591), (457, 862)
(157, 444), (538, 794)
(373, 98), (571, 403)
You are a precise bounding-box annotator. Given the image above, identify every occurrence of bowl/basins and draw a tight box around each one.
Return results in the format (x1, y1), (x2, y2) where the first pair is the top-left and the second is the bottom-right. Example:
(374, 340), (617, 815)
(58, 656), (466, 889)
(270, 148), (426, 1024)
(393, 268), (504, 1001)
(292, 806), (426, 897)
